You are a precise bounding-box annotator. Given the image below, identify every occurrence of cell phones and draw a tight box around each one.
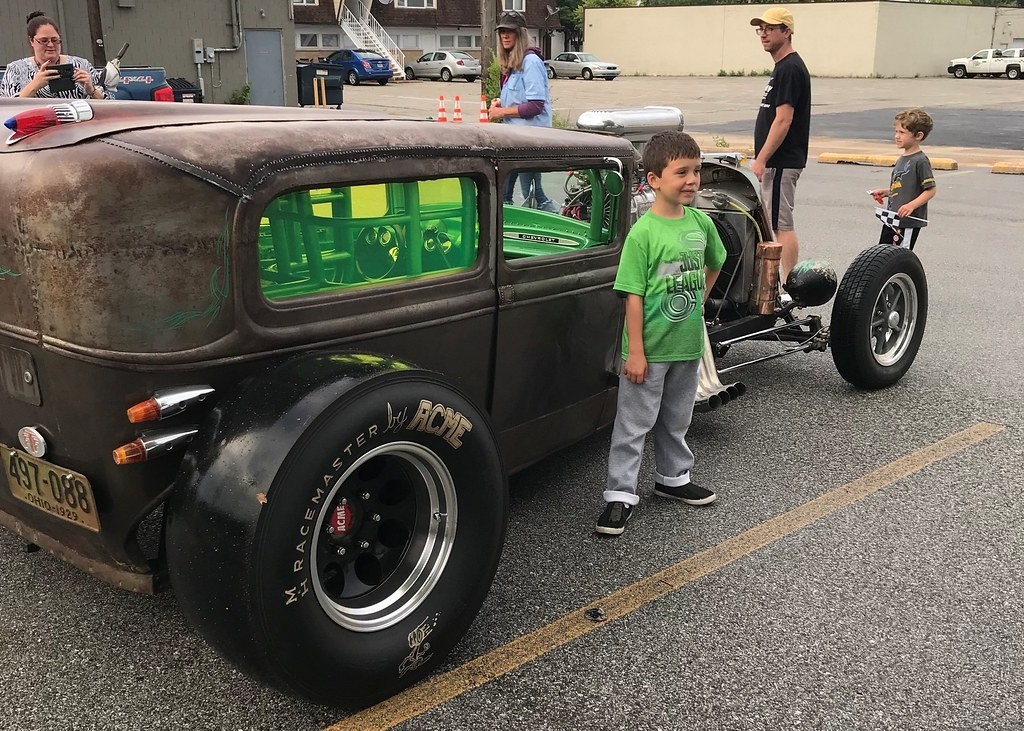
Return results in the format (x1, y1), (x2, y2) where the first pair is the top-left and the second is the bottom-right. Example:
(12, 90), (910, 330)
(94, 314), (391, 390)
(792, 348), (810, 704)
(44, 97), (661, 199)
(46, 62), (76, 93)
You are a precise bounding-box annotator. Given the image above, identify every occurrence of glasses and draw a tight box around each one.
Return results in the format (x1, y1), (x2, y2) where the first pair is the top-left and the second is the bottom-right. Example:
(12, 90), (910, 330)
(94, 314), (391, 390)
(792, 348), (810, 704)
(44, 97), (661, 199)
(33, 36), (62, 45)
(500, 10), (526, 26)
(756, 25), (782, 35)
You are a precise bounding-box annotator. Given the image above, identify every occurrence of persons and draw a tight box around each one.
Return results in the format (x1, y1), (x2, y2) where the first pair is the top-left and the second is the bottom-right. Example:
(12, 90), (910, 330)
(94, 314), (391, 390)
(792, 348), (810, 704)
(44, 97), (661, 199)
(749, 7), (812, 304)
(486, 10), (562, 215)
(594, 131), (727, 536)
(0, 9), (115, 100)
(873, 108), (936, 252)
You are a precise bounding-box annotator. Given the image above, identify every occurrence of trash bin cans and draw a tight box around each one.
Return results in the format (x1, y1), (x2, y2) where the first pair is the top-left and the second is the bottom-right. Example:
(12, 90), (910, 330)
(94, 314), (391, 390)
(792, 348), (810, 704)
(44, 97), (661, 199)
(297, 61), (345, 112)
(167, 78), (205, 103)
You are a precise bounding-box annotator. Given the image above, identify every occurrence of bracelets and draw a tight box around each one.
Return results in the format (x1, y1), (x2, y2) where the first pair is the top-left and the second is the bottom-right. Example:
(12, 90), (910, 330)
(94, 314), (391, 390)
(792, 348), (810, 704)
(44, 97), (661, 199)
(87, 85), (97, 98)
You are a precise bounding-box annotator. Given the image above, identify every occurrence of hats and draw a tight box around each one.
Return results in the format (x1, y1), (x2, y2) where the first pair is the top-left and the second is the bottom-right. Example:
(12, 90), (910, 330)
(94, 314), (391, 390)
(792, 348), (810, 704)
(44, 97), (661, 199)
(750, 7), (794, 34)
(494, 11), (528, 31)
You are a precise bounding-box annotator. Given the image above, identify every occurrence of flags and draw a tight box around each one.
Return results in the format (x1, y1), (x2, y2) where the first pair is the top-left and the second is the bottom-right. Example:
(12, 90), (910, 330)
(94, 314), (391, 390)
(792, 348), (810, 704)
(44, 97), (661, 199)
(874, 209), (904, 239)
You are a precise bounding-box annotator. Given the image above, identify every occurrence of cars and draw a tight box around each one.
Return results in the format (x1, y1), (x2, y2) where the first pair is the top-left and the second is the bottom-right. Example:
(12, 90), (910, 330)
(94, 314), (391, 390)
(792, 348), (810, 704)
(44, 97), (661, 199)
(327, 48), (393, 86)
(404, 49), (482, 83)
(0, 103), (930, 711)
(544, 52), (622, 81)
(1001, 47), (1024, 58)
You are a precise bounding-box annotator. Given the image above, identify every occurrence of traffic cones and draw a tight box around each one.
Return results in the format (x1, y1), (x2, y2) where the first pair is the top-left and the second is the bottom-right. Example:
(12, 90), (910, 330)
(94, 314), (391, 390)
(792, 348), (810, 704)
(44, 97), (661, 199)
(452, 95), (464, 122)
(438, 95), (448, 122)
(478, 95), (489, 123)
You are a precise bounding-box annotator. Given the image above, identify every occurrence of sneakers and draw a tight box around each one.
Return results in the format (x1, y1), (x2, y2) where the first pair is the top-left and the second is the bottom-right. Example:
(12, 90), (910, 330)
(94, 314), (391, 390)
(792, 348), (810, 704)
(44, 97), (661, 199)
(595, 501), (634, 535)
(654, 482), (716, 506)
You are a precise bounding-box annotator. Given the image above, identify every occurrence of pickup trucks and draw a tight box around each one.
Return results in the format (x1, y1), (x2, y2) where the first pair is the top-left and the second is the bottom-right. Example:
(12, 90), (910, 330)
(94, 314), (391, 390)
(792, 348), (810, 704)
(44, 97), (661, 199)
(0, 65), (176, 103)
(946, 48), (1024, 79)
